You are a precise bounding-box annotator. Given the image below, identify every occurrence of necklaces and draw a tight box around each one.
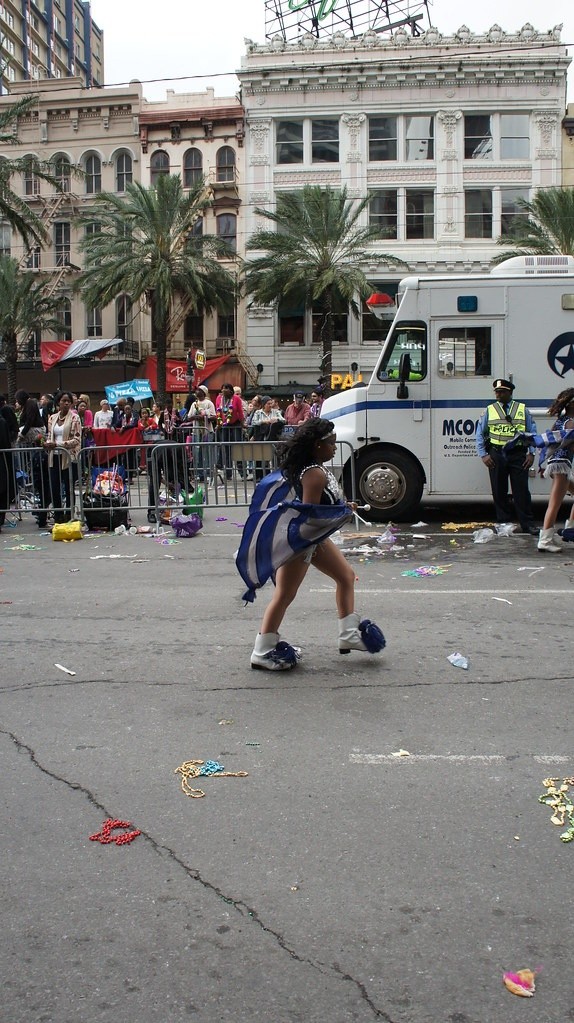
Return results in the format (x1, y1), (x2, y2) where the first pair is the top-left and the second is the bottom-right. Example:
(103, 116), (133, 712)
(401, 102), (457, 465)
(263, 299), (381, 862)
(217, 396), (233, 426)
(59, 415), (65, 421)
(223, 397), (229, 412)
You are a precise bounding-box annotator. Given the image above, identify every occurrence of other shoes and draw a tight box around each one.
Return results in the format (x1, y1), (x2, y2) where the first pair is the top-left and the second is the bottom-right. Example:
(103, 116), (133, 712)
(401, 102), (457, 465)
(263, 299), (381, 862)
(222, 476), (233, 483)
(124, 477), (134, 485)
(522, 523), (540, 535)
(241, 476), (245, 481)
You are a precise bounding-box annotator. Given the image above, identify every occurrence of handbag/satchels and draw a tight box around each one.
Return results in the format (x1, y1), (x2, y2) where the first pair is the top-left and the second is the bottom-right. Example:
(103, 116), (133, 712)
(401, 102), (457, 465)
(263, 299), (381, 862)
(158, 484), (205, 538)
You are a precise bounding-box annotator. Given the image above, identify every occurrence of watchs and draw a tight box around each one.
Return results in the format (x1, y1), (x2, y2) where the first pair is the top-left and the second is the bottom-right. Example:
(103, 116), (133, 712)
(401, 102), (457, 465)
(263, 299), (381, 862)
(530, 452), (535, 456)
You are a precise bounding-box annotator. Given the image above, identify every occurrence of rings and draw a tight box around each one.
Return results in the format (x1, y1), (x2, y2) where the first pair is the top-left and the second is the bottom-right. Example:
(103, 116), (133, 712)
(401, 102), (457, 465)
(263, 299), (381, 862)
(351, 502), (354, 503)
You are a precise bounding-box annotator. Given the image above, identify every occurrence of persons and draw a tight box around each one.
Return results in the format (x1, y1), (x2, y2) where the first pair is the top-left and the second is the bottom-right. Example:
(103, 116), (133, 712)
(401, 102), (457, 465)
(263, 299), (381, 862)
(33, 392), (81, 529)
(0, 383), (325, 485)
(476, 379), (541, 536)
(0, 404), (19, 525)
(147, 439), (189, 522)
(502, 387), (574, 553)
(235, 417), (387, 671)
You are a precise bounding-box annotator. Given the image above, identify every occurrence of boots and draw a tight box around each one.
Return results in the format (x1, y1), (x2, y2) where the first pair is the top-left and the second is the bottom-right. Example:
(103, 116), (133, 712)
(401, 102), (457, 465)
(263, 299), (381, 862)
(338, 613), (386, 654)
(537, 526), (563, 553)
(251, 632), (301, 672)
(562, 520), (574, 549)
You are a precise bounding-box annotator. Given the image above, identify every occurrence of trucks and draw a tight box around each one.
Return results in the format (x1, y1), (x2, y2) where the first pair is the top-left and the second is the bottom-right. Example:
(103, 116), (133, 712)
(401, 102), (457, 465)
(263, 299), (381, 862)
(319, 253), (574, 524)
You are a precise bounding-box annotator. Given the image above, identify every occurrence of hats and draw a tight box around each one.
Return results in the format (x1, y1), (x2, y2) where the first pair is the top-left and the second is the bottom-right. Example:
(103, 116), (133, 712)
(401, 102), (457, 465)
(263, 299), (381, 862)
(261, 396), (271, 406)
(493, 379), (515, 392)
(198, 385), (208, 395)
(295, 390), (306, 402)
(100, 399), (109, 406)
